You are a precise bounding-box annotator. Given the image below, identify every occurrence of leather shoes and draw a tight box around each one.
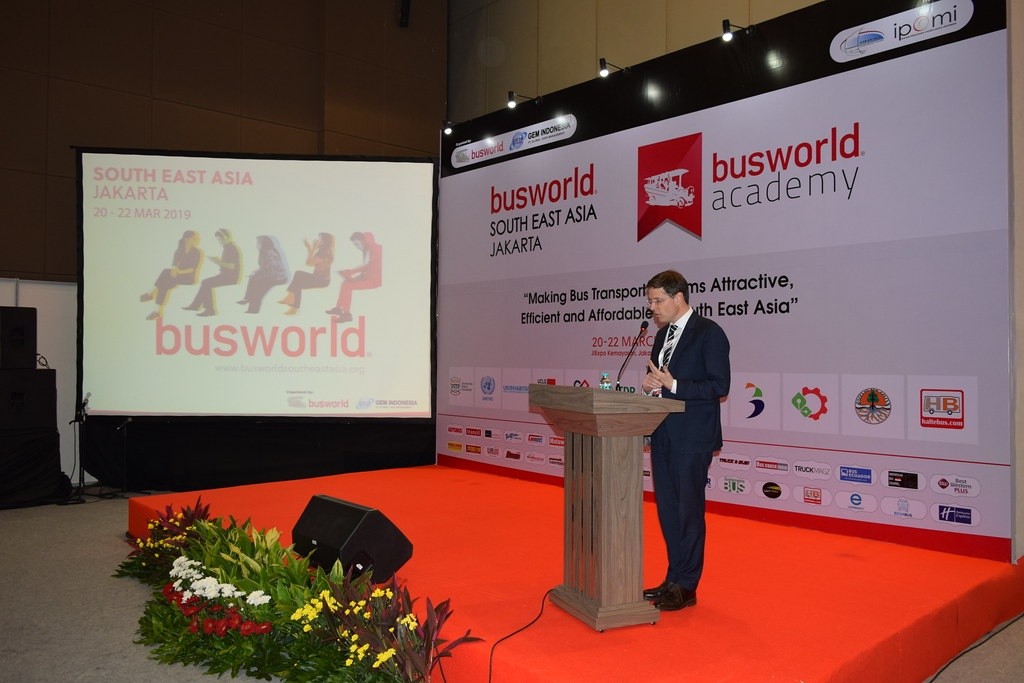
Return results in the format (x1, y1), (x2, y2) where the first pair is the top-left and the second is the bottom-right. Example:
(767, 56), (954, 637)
(654, 583), (697, 610)
(643, 579), (674, 599)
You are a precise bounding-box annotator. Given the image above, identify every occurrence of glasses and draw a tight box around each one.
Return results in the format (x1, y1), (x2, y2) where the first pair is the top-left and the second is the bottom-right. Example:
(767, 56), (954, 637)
(648, 295), (676, 304)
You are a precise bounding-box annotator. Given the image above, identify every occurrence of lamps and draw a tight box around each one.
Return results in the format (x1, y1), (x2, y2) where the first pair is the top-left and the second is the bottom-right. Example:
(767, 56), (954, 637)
(722, 19), (745, 42)
(507, 90), (532, 109)
(599, 57), (624, 78)
(442, 119), (460, 134)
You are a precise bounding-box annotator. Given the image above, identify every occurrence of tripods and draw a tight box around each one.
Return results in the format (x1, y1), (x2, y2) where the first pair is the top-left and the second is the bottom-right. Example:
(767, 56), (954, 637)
(73, 408), (151, 496)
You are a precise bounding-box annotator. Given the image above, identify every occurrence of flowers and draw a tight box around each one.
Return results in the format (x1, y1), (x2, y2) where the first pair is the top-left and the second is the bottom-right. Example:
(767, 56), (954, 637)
(110, 495), (486, 683)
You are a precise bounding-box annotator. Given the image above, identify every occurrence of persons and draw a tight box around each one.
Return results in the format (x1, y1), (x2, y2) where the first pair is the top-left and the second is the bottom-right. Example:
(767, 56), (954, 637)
(642, 267), (732, 611)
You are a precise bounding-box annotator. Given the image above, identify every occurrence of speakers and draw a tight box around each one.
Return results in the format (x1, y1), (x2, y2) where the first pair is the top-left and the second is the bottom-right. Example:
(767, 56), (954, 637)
(0, 306), (37, 368)
(0, 368), (58, 437)
(291, 494), (413, 582)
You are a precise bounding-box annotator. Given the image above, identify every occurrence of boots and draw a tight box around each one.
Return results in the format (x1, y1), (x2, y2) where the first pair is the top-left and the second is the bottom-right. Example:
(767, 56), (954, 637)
(278, 292), (294, 305)
(286, 305), (301, 315)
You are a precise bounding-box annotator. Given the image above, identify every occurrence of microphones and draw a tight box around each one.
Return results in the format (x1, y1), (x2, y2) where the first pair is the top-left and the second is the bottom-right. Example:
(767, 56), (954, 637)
(615, 321), (648, 391)
(77, 399), (88, 414)
(117, 418), (132, 429)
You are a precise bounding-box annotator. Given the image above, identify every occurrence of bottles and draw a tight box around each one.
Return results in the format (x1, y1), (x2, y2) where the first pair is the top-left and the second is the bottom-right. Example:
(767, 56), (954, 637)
(598, 372), (612, 390)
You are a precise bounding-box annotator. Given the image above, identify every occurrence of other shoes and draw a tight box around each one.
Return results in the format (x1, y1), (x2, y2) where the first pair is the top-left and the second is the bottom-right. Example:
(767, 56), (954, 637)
(245, 308), (260, 314)
(334, 313), (354, 324)
(196, 309), (217, 317)
(236, 297), (250, 304)
(147, 311), (160, 321)
(327, 306), (342, 315)
(140, 294), (152, 302)
(181, 304), (197, 310)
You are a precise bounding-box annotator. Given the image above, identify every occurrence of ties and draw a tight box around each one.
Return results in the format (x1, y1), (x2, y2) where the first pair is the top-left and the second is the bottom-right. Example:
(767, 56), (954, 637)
(659, 325), (678, 373)
